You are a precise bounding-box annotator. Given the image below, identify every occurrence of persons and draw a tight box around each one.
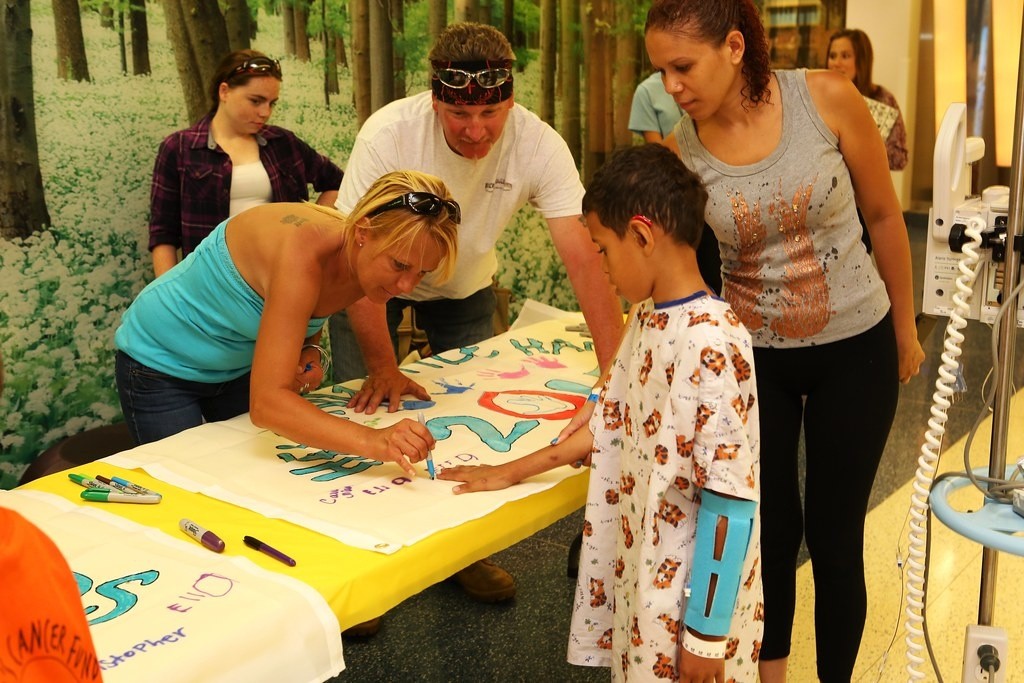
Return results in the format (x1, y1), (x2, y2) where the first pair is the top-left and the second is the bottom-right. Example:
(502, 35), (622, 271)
(564, 141), (769, 683)
(147, 49), (345, 416)
(619, 1), (926, 681)
(330, 26), (626, 644)
(113, 172), (465, 481)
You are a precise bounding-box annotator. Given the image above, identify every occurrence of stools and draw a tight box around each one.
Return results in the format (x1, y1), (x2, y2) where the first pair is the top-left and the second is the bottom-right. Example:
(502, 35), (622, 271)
(18, 424), (137, 488)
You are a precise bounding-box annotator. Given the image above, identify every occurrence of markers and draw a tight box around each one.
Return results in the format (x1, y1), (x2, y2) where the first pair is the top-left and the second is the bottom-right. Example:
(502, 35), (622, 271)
(178, 518), (226, 553)
(80, 486), (161, 505)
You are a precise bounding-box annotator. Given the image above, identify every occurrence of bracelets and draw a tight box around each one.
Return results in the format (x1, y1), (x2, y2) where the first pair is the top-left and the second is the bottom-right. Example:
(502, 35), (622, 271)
(302, 343), (330, 376)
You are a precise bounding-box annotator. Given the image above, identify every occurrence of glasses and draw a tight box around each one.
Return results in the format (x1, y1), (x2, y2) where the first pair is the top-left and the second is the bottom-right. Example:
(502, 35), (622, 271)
(368, 191), (463, 226)
(433, 67), (511, 89)
(233, 57), (281, 75)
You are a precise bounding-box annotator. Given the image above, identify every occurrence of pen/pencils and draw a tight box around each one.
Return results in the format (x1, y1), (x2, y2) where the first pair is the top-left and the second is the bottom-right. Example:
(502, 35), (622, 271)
(416, 411), (435, 481)
(242, 535), (298, 568)
(67, 472), (163, 499)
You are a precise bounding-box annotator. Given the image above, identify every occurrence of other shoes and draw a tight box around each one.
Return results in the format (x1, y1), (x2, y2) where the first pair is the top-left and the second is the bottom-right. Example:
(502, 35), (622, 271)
(457, 559), (517, 603)
(342, 618), (384, 637)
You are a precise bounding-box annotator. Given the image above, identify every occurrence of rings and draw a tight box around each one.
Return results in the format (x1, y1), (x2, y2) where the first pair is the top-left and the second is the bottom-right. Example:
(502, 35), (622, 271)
(301, 383), (310, 392)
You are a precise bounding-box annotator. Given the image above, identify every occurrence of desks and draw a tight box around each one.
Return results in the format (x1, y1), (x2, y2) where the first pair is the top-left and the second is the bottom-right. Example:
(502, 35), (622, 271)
(20, 460), (591, 631)
